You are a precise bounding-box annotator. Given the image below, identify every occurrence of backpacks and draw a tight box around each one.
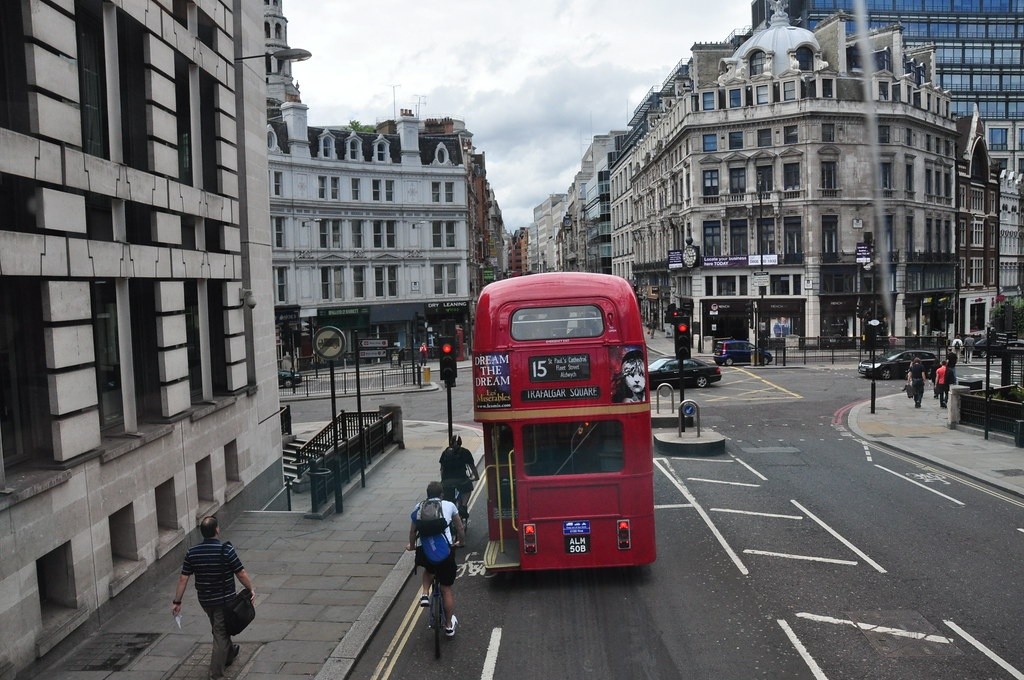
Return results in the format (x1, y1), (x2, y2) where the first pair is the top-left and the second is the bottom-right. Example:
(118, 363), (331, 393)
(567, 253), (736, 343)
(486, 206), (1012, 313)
(954, 339), (960, 349)
(420, 498), (448, 535)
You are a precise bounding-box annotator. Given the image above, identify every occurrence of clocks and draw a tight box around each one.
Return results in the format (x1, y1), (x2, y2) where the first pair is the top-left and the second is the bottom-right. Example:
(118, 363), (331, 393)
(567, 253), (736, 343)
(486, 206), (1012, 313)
(682, 247), (696, 265)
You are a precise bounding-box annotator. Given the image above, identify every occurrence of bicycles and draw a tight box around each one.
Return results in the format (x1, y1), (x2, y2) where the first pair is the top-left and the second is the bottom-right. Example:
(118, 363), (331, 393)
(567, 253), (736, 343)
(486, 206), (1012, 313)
(446, 473), (479, 549)
(408, 544), (464, 659)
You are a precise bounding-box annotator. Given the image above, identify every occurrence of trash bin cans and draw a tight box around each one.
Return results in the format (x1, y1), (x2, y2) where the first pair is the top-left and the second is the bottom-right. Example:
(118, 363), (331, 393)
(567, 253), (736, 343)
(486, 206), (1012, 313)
(379, 404), (404, 444)
(958, 379), (984, 393)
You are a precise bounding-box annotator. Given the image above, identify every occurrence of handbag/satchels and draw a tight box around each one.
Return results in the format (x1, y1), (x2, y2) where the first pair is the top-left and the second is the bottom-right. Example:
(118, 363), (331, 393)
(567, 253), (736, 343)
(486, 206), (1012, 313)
(221, 589), (256, 635)
(905, 384), (913, 398)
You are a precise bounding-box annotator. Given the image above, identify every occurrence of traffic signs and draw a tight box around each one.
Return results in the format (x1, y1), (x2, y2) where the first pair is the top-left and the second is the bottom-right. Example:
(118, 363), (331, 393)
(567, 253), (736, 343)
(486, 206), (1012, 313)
(359, 339), (389, 348)
(358, 350), (387, 359)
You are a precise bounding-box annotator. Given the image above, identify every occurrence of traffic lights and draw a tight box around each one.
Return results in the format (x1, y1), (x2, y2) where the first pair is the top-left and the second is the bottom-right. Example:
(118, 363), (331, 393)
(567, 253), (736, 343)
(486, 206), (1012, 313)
(673, 309), (683, 318)
(674, 315), (691, 359)
(440, 335), (456, 380)
(305, 316), (312, 340)
(414, 311), (425, 333)
(279, 322), (286, 342)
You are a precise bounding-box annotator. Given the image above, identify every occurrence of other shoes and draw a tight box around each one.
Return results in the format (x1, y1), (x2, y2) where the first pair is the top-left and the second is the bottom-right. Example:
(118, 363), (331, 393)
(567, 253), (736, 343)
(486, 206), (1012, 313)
(940, 400), (948, 408)
(458, 505), (469, 518)
(916, 401), (921, 408)
(225, 645), (240, 666)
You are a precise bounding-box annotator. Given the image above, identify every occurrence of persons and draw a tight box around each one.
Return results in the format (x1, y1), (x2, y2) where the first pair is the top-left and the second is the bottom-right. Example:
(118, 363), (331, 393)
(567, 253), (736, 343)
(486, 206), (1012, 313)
(951, 335), (963, 362)
(929, 356), (953, 408)
(405, 481), (466, 637)
(438, 434), (479, 523)
(420, 343), (428, 366)
(905, 357), (926, 408)
(946, 347), (957, 380)
(171, 517), (256, 680)
(964, 335), (975, 363)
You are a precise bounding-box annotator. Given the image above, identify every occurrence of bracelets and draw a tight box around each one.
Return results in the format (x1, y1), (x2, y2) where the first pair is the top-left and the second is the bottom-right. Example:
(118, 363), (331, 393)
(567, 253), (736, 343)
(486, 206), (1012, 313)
(173, 601), (181, 604)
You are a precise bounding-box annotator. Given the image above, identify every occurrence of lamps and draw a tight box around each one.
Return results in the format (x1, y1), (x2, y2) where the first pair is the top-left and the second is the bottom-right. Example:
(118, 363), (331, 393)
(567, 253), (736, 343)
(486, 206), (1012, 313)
(235, 48), (312, 61)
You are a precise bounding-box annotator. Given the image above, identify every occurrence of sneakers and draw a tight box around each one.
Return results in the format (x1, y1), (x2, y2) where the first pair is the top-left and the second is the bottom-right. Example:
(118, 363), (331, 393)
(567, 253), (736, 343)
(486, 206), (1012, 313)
(445, 613), (459, 635)
(420, 594), (430, 607)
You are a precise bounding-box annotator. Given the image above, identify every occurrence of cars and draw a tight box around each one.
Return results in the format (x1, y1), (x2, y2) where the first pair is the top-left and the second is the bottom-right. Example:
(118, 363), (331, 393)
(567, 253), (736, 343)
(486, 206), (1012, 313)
(858, 349), (941, 380)
(278, 368), (303, 388)
(648, 356), (722, 391)
(714, 340), (773, 367)
(961, 334), (1024, 359)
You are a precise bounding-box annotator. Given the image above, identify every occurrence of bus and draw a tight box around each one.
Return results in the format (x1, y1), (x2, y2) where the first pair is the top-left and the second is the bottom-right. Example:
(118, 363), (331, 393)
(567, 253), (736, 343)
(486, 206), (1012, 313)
(469, 270), (658, 576)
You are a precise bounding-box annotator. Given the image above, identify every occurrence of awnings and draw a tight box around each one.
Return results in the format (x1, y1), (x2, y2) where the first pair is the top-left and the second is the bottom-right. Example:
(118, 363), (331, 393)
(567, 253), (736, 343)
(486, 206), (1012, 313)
(370, 303), (426, 324)
